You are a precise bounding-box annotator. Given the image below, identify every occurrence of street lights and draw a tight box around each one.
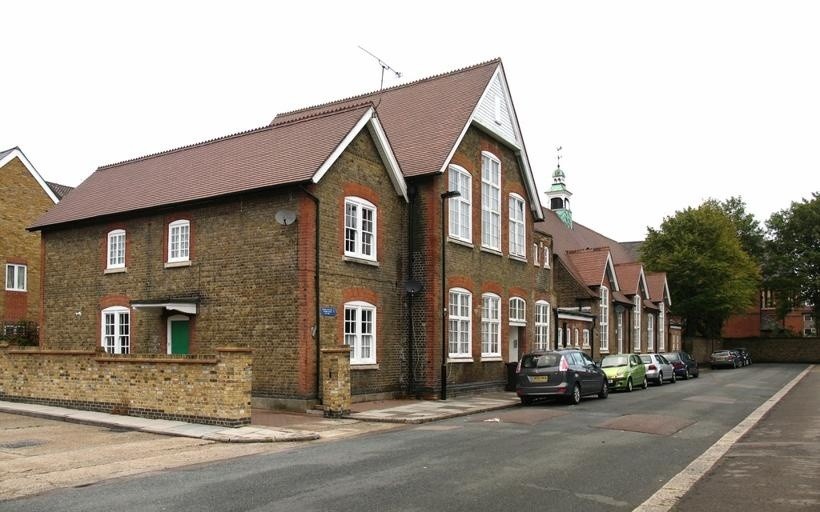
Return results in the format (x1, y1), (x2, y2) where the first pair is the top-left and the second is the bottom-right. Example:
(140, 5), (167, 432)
(440, 189), (462, 400)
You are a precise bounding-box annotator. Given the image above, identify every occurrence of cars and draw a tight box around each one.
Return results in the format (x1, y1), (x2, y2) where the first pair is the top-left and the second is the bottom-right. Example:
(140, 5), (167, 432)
(638, 353), (677, 384)
(709, 346), (752, 371)
(660, 350), (700, 379)
(514, 349), (608, 404)
(600, 353), (648, 392)
